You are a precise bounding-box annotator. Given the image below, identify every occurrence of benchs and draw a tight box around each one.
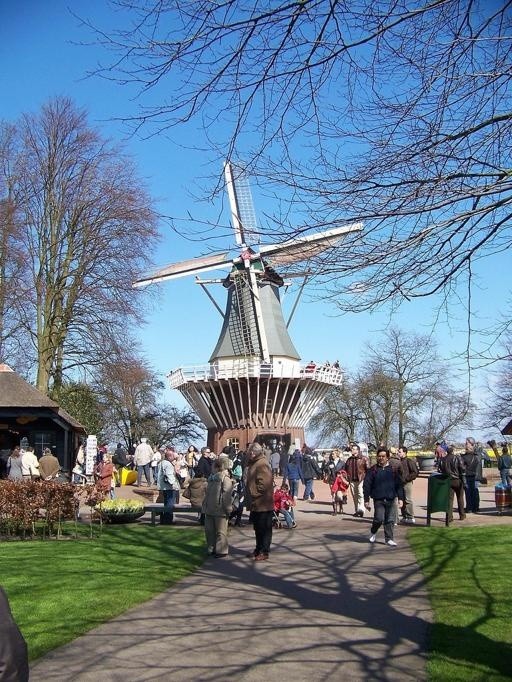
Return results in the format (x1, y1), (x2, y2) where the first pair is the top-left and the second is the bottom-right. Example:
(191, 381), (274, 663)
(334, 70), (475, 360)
(143, 503), (202, 526)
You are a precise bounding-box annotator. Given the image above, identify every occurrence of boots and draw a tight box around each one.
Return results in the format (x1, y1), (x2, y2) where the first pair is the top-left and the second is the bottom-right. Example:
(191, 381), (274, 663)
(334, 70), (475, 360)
(339, 503), (343, 514)
(332, 502), (338, 516)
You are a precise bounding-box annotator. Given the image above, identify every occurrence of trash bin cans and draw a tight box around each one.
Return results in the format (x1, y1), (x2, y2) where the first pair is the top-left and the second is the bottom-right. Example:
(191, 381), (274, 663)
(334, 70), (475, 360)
(426, 472), (450, 527)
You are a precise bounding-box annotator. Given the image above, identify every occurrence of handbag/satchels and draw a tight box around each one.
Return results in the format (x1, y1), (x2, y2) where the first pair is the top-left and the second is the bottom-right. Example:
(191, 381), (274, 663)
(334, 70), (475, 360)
(182, 488), (191, 499)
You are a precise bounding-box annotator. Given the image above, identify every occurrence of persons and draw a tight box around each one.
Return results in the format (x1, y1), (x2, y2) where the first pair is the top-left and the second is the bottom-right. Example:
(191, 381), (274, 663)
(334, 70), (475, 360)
(242, 442), (276, 561)
(1, 585), (30, 681)
(497, 444), (511, 487)
(362, 447), (405, 547)
(199, 455), (234, 559)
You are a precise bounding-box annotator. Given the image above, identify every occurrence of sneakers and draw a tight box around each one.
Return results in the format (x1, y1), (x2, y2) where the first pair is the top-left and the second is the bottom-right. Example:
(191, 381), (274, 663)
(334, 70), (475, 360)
(460, 514), (466, 520)
(448, 518), (454, 523)
(386, 540), (398, 547)
(369, 532), (376, 543)
(353, 512), (364, 517)
(247, 552), (269, 561)
(400, 519), (407, 524)
(407, 519), (416, 524)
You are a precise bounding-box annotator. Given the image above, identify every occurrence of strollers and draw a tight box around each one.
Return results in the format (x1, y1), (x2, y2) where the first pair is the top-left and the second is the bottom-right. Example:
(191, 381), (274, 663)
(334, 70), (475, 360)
(271, 492), (297, 529)
